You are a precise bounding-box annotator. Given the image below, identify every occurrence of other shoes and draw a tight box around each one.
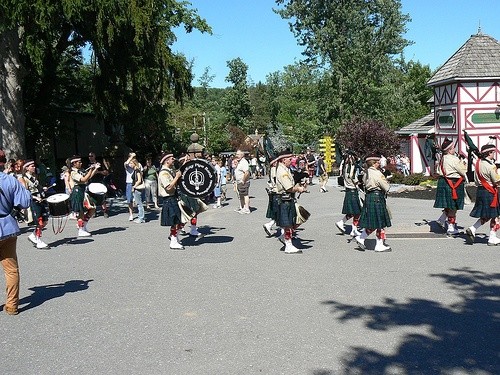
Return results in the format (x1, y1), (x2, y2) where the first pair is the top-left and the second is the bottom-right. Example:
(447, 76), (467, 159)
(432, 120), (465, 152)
(263, 222), (273, 236)
(446, 222), (464, 236)
(374, 240), (392, 253)
(27, 232), (49, 249)
(334, 219), (367, 251)
(0, 303), (18, 315)
(487, 231), (500, 246)
(278, 233), (303, 254)
(188, 224), (201, 236)
(466, 225), (477, 244)
(133, 217), (145, 224)
(436, 211), (448, 231)
(213, 204), (223, 209)
(234, 206), (251, 214)
(78, 230), (92, 237)
(76, 221), (90, 233)
(168, 234), (184, 249)
(128, 214), (133, 221)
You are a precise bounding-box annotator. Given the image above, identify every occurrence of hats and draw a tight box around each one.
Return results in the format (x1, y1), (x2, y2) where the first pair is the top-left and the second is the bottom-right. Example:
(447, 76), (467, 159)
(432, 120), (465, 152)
(268, 146), (293, 166)
(364, 151), (381, 162)
(480, 143), (497, 153)
(160, 149), (174, 165)
(441, 136), (452, 150)
(69, 154), (83, 164)
(20, 159), (34, 170)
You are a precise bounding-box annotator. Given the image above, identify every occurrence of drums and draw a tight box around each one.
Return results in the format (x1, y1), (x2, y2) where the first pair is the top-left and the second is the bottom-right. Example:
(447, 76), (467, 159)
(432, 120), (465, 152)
(45, 192), (73, 218)
(176, 158), (219, 199)
(85, 182), (108, 207)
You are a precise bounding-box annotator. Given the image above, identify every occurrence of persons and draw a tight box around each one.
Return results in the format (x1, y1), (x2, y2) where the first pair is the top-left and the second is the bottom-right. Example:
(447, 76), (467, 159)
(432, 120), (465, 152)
(466, 144), (500, 246)
(262, 153), (294, 236)
(157, 152), (208, 251)
(433, 136), (469, 235)
(60, 158), (79, 220)
(211, 150), (329, 212)
(377, 153), (411, 180)
(85, 152), (113, 218)
(234, 149), (251, 215)
(20, 159), (50, 249)
(130, 159), (146, 224)
(143, 159), (159, 209)
(0, 149), (32, 315)
(68, 153), (101, 237)
(121, 152), (144, 221)
(355, 154), (393, 252)
(277, 150), (307, 254)
(335, 154), (365, 236)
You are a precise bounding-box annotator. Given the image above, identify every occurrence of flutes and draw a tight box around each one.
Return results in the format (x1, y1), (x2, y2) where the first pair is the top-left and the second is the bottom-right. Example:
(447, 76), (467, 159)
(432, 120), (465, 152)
(254, 134), (310, 198)
(425, 135), (466, 181)
(463, 129), (500, 182)
(333, 140), (392, 196)
